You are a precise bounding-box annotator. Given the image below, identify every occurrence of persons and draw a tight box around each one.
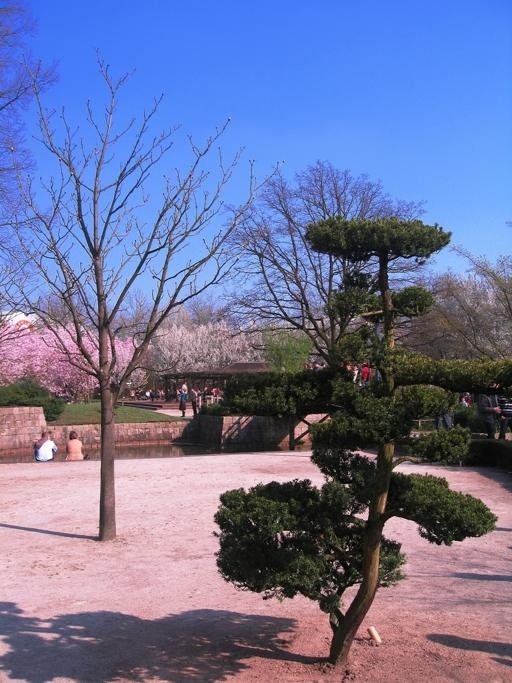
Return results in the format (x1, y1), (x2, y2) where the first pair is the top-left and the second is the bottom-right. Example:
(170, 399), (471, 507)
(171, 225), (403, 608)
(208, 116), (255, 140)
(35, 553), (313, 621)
(460, 390), (473, 408)
(64, 430), (86, 462)
(479, 380), (502, 440)
(498, 392), (512, 440)
(303, 358), (381, 392)
(128, 378), (219, 418)
(32, 431), (59, 464)
